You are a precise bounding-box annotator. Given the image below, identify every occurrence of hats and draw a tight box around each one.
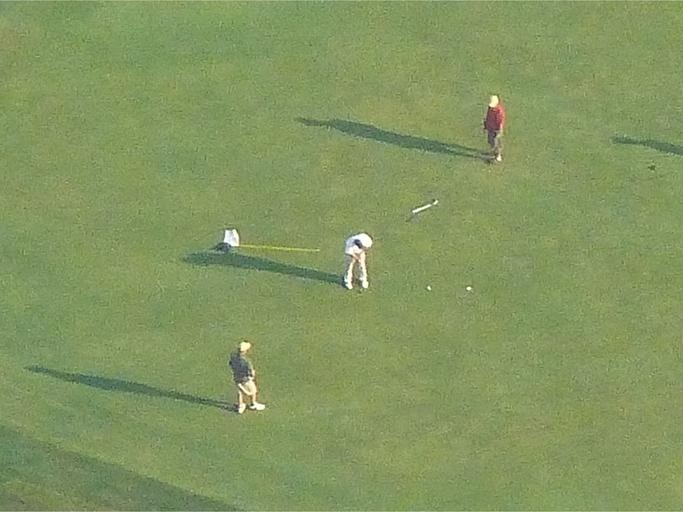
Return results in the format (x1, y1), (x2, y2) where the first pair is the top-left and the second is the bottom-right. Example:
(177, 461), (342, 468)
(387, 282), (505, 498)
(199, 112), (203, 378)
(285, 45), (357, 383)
(239, 342), (251, 351)
(488, 95), (499, 107)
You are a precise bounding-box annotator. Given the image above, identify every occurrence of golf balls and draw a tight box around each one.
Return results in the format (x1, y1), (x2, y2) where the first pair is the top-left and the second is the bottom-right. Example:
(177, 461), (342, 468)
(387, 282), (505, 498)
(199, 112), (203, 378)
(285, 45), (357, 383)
(426, 286), (432, 292)
(466, 286), (472, 291)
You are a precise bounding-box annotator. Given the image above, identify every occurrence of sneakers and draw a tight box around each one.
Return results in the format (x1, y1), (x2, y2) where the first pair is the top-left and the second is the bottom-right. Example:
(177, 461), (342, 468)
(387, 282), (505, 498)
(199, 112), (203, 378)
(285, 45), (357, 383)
(345, 282), (369, 290)
(238, 402), (266, 414)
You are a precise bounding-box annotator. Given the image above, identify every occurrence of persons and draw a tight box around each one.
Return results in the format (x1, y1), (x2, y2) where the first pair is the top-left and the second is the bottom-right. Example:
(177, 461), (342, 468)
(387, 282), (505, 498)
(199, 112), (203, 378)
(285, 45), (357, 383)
(483, 95), (505, 162)
(228, 341), (264, 414)
(342, 231), (373, 290)
